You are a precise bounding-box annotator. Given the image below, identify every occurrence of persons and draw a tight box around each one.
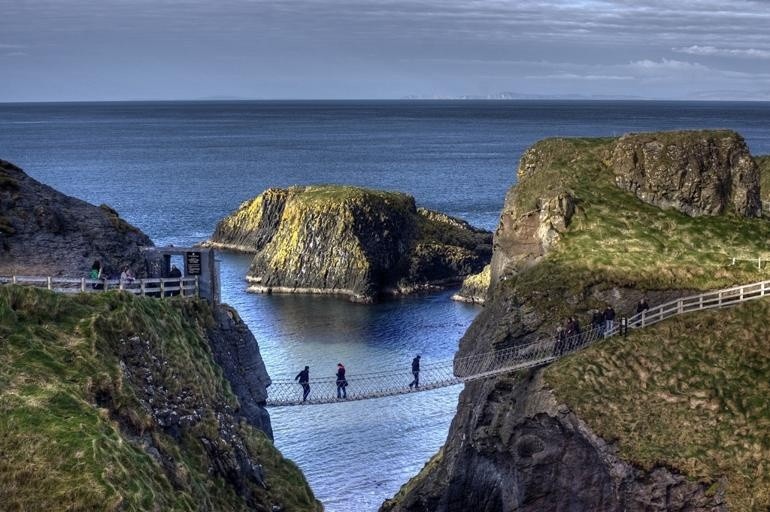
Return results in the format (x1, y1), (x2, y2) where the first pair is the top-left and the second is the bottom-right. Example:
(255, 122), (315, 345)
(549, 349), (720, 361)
(119, 266), (135, 291)
(294, 365), (311, 402)
(168, 264), (182, 296)
(589, 304), (616, 340)
(550, 314), (583, 357)
(89, 260), (105, 289)
(635, 296), (649, 328)
(409, 355), (421, 389)
(336, 363), (348, 401)
